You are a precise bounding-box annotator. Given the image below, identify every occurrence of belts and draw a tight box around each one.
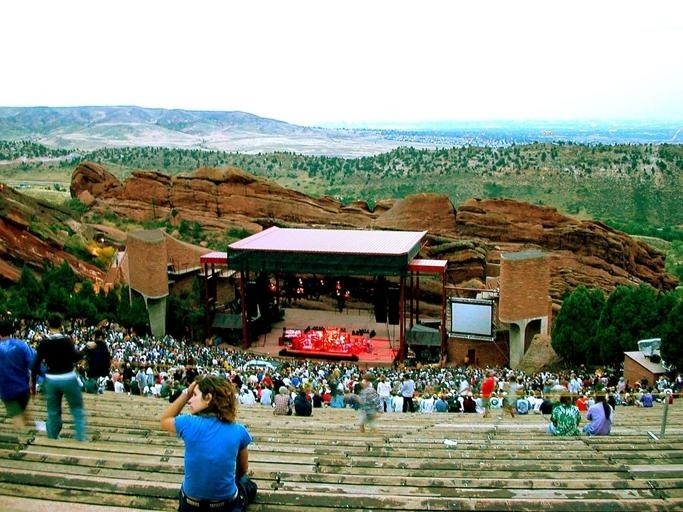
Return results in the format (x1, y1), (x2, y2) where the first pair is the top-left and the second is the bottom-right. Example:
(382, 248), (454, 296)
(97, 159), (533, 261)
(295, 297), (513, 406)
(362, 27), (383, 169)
(182, 488), (238, 508)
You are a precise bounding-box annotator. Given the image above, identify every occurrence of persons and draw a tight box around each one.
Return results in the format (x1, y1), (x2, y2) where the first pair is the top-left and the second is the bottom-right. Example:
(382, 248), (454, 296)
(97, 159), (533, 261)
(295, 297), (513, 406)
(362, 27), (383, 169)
(30, 315), (86, 441)
(8, 311), (682, 416)
(357, 372), (378, 434)
(160, 375), (257, 512)
(548, 391), (582, 437)
(0, 321), (46, 459)
(582, 390), (615, 435)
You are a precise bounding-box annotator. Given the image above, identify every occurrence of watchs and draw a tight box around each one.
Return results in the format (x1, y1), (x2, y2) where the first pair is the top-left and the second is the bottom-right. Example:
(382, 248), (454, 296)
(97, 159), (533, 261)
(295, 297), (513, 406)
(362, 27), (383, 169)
(182, 388), (192, 399)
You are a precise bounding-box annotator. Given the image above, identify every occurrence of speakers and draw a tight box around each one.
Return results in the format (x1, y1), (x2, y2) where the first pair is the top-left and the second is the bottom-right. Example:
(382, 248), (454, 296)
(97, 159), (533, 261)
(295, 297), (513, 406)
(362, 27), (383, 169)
(388, 288), (400, 325)
(246, 276), (272, 321)
(373, 274), (387, 322)
